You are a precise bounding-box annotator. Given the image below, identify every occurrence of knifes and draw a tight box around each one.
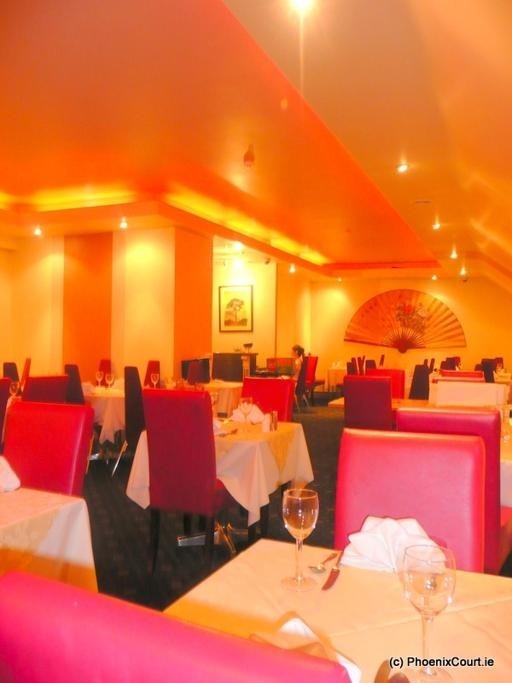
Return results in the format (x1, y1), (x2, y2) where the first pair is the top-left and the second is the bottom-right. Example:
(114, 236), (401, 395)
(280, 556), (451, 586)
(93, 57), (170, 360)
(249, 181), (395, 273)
(321, 551), (344, 591)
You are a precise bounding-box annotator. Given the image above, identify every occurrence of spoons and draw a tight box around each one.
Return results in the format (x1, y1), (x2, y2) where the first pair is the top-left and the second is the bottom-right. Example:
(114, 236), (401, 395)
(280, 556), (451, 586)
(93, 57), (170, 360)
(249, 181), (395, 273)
(308, 553), (338, 574)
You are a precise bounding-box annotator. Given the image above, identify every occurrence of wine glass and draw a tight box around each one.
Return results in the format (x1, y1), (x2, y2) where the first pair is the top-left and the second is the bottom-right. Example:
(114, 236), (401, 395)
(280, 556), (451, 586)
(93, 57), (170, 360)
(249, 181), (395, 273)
(9, 382), (20, 398)
(96, 370), (104, 386)
(238, 397), (254, 429)
(398, 542), (457, 683)
(105, 374), (114, 387)
(151, 373), (160, 387)
(279, 487), (321, 590)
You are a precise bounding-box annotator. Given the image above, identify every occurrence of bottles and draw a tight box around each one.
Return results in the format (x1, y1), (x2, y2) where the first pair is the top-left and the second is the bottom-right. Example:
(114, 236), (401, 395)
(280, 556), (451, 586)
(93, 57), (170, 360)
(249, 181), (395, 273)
(270, 410), (277, 431)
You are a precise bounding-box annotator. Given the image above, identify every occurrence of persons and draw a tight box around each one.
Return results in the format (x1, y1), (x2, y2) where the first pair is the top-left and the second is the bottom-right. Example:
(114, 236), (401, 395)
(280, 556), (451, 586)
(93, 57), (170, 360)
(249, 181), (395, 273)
(290, 343), (307, 394)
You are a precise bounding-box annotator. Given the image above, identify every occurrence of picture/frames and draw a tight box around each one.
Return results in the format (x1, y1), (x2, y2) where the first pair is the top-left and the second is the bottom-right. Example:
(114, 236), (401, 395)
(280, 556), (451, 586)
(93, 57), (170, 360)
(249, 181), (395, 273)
(218, 284), (253, 332)
